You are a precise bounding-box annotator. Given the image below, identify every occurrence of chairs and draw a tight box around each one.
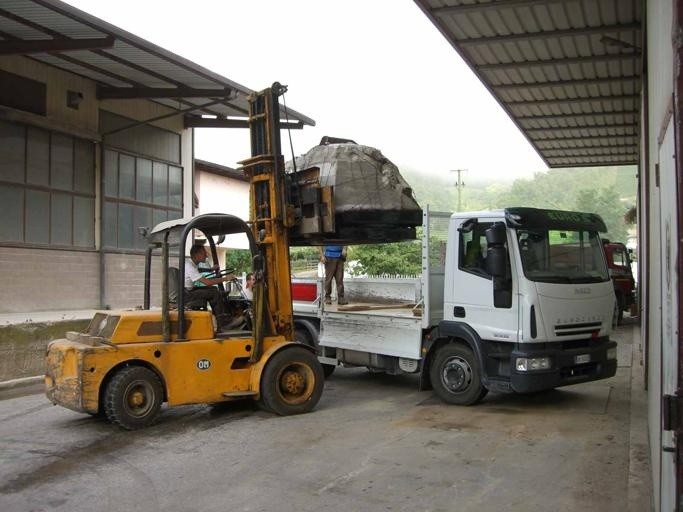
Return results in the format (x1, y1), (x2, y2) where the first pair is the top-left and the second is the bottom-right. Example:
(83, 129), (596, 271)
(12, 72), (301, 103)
(516, 238), (541, 271)
(484, 227), (510, 283)
(164, 267), (210, 312)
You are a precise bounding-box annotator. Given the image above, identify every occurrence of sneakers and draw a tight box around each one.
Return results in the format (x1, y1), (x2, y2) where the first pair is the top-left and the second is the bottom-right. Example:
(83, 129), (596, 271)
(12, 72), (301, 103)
(217, 316), (244, 330)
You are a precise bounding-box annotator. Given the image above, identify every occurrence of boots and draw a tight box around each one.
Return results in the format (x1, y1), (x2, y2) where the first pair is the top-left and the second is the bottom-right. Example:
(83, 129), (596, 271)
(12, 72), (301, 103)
(325, 295), (331, 304)
(338, 294), (349, 305)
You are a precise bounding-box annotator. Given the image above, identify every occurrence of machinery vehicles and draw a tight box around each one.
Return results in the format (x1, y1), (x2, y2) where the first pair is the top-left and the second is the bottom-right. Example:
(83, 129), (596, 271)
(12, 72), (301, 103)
(42, 82), (423, 433)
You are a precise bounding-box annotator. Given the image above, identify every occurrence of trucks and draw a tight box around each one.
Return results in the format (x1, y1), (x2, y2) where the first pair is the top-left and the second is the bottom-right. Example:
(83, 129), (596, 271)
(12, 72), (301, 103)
(439, 240), (638, 327)
(237, 205), (619, 410)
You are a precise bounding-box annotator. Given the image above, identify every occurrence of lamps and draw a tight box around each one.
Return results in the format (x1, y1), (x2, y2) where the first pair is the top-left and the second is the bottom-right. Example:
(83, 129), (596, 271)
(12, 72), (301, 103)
(597, 35), (641, 53)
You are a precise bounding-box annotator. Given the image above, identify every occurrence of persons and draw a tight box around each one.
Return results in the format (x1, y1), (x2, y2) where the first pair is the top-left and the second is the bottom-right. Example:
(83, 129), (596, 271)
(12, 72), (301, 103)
(316, 244), (349, 306)
(183, 244), (244, 330)
(238, 272), (256, 302)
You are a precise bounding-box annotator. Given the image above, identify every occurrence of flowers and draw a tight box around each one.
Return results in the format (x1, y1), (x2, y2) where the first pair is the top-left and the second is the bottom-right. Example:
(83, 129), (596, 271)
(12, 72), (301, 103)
(620, 204), (636, 225)
